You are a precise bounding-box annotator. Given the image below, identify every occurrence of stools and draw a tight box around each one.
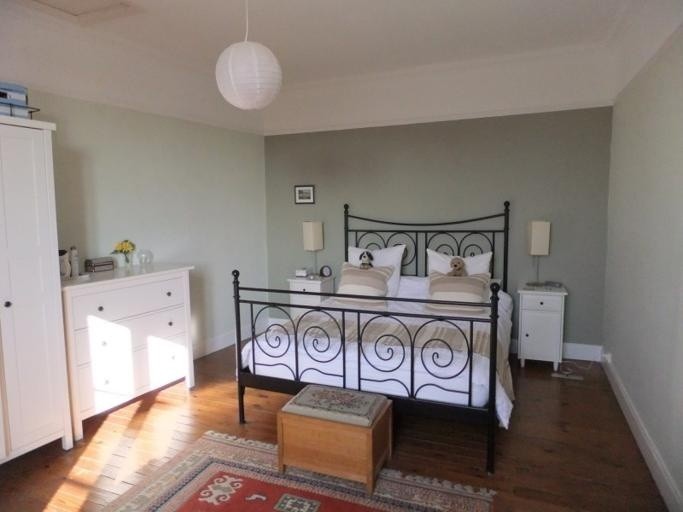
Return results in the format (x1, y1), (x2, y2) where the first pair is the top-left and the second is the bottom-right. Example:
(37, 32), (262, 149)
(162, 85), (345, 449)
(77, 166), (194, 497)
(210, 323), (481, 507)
(274, 381), (397, 498)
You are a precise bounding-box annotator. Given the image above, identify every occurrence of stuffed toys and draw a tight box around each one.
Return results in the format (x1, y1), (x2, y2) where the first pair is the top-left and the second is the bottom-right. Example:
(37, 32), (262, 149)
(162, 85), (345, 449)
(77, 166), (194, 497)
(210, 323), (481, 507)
(448, 257), (467, 277)
(358, 251), (373, 269)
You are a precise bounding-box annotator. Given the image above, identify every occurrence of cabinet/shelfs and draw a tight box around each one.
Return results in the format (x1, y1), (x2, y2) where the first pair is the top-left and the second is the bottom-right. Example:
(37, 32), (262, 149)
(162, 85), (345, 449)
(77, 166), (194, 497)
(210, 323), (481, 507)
(0, 113), (76, 469)
(60, 263), (200, 440)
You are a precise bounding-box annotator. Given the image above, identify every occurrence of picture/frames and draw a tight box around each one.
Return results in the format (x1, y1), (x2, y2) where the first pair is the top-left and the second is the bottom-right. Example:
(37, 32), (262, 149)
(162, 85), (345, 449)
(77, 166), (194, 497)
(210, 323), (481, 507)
(293, 184), (317, 205)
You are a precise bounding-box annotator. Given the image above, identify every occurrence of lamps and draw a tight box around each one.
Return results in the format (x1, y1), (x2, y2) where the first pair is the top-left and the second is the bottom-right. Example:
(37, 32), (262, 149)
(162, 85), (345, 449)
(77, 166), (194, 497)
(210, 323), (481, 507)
(525, 219), (553, 290)
(215, 0), (286, 114)
(301, 219), (326, 275)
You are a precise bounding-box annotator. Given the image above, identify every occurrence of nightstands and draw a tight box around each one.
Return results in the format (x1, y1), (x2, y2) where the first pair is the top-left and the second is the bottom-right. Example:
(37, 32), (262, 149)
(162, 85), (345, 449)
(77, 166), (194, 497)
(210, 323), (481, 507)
(515, 281), (570, 372)
(286, 276), (335, 321)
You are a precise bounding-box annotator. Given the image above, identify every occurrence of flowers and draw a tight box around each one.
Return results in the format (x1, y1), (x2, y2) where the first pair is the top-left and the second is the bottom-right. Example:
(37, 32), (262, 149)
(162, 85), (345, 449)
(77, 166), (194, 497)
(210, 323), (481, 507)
(113, 238), (135, 252)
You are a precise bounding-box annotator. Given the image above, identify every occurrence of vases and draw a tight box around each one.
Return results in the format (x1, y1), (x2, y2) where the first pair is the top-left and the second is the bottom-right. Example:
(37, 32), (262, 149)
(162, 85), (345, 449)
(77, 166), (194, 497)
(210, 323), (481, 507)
(113, 253), (128, 267)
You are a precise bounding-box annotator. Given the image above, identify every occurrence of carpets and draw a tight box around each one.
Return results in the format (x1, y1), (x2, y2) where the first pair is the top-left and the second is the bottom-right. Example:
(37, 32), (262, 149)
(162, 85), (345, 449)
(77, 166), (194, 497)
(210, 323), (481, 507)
(95, 426), (499, 512)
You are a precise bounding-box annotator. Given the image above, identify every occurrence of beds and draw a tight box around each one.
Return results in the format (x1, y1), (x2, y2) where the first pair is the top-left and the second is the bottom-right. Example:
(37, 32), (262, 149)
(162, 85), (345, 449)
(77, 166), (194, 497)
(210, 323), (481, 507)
(231, 199), (517, 473)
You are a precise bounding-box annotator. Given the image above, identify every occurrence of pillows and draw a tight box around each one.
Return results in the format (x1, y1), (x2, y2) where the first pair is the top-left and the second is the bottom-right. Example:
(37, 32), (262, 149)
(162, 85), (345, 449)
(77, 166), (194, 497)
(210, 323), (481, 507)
(424, 267), (493, 317)
(346, 242), (408, 305)
(426, 247), (494, 286)
(334, 261), (394, 315)
